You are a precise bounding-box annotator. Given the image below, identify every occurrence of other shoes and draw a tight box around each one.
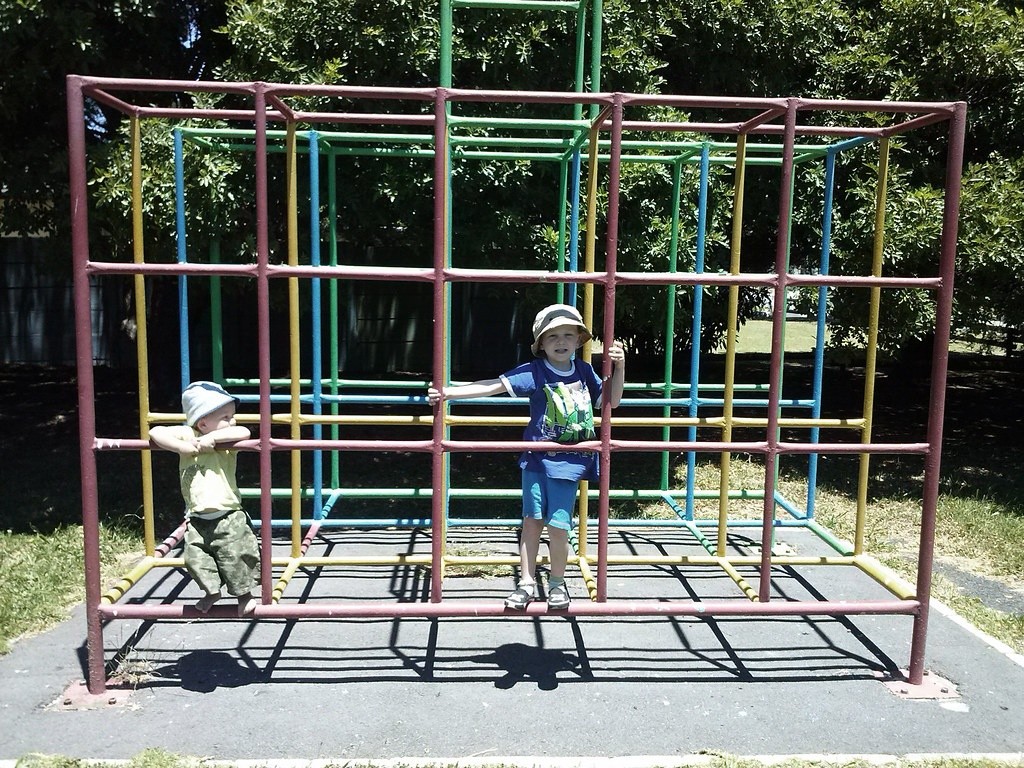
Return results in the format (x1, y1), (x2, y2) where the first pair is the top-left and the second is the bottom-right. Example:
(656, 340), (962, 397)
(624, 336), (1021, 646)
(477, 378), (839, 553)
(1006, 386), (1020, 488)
(504, 579), (541, 611)
(547, 576), (572, 610)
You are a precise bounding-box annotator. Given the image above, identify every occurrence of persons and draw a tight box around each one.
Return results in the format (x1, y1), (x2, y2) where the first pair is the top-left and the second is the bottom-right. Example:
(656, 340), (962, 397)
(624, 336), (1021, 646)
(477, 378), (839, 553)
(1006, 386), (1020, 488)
(148, 381), (262, 618)
(428, 303), (626, 611)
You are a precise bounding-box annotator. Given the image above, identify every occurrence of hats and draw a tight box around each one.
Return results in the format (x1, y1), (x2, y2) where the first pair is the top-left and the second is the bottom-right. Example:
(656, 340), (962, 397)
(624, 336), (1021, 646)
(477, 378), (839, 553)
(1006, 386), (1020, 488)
(530, 304), (596, 359)
(181, 381), (242, 429)
